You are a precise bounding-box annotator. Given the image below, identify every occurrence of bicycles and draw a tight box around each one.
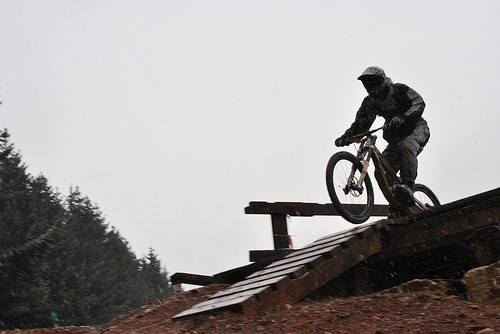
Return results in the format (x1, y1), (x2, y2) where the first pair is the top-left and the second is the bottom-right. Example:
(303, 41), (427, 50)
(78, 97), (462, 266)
(326, 119), (440, 224)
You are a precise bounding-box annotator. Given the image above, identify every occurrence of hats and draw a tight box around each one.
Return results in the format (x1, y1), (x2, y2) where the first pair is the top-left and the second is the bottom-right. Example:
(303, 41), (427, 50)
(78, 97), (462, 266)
(356, 65), (383, 80)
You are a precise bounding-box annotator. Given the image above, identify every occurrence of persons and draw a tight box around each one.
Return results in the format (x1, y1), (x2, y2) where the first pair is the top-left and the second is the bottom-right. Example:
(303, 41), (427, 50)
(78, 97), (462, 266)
(335, 67), (431, 218)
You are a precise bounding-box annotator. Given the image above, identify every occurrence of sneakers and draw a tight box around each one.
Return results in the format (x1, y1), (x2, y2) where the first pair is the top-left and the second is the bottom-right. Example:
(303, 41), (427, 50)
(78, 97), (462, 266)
(387, 206), (401, 219)
(393, 182), (417, 207)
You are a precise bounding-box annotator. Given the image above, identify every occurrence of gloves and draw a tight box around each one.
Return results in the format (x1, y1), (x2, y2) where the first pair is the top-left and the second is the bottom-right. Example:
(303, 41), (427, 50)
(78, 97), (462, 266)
(388, 116), (406, 129)
(335, 133), (352, 149)
